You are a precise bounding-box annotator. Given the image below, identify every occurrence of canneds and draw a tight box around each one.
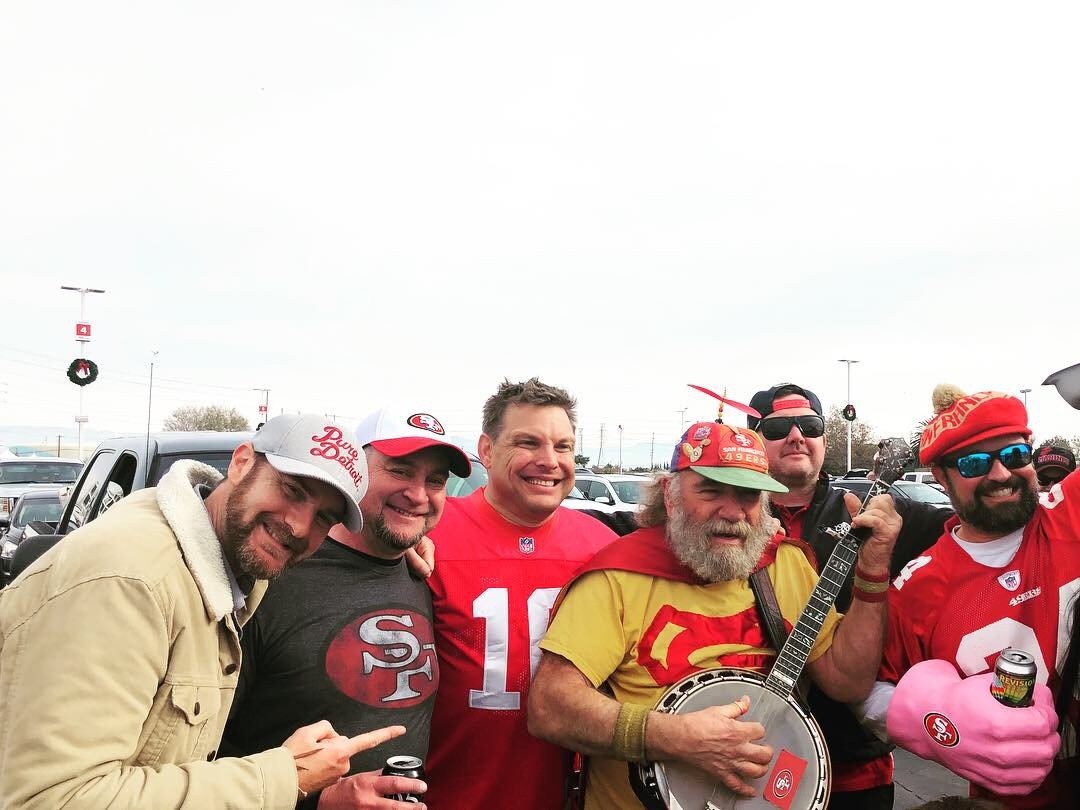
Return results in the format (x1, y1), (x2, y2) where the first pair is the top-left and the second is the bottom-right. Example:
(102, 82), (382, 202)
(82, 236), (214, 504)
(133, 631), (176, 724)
(990, 649), (1037, 709)
(382, 755), (425, 803)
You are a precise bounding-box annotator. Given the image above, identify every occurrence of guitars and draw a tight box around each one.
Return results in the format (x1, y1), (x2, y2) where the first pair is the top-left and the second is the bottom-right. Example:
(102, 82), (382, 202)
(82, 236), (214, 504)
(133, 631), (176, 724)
(626, 435), (916, 810)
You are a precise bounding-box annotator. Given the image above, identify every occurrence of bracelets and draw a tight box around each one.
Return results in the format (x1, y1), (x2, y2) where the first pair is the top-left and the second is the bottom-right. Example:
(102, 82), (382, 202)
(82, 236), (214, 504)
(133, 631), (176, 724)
(853, 565), (890, 604)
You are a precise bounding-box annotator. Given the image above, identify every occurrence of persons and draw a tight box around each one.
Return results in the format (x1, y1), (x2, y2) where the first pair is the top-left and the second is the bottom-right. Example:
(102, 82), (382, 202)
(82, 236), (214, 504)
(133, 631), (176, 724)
(0, 376), (1079, 810)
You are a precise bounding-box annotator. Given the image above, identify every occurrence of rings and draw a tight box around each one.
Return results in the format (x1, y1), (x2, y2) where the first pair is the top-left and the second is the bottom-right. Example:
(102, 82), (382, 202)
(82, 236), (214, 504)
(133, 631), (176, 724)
(733, 698), (749, 715)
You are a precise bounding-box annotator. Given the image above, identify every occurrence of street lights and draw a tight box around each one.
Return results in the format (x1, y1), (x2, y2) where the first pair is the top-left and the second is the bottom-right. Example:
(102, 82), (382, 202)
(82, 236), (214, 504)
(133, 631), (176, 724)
(837, 359), (860, 475)
(253, 388), (272, 423)
(619, 424), (623, 475)
(676, 410), (685, 435)
(1019, 388), (1031, 407)
(59, 285), (106, 460)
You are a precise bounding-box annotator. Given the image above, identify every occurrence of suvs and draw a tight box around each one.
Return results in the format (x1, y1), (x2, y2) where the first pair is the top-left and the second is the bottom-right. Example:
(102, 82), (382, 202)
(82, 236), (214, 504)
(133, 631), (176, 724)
(10, 431), (256, 581)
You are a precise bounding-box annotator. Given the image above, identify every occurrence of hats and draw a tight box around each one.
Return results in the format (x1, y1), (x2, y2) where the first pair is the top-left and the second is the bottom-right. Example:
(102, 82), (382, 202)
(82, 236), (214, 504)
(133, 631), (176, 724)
(356, 403), (472, 479)
(670, 422), (790, 494)
(250, 413), (368, 534)
(1033, 447), (1077, 474)
(747, 382), (822, 431)
(919, 383), (1033, 465)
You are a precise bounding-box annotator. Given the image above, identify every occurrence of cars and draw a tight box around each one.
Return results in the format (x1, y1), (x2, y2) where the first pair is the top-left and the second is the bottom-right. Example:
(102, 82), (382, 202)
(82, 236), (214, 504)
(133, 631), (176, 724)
(445, 450), (488, 499)
(827, 468), (955, 511)
(0, 445), (83, 590)
(559, 467), (670, 514)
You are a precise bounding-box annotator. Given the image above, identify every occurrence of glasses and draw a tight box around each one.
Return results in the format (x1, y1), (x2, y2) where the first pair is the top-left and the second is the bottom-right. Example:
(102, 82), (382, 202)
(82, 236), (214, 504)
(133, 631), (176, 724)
(938, 441), (1033, 479)
(1037, 474), (1064, 488)
(754, 415), (825, 441)
